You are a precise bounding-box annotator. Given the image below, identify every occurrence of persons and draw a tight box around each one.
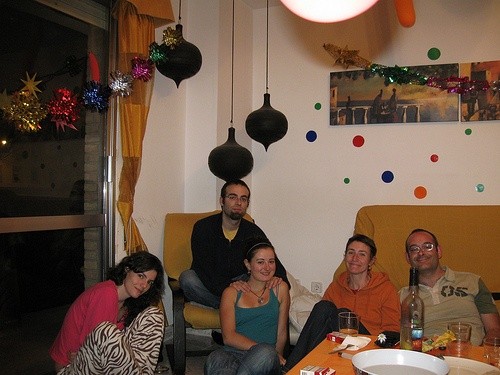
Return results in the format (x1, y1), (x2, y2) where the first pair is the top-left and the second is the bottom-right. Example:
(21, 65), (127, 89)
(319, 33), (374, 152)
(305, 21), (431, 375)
(50, 250), (165, 375)
(179, 178), (291, 308)
(204, 239), (290, 375)
(398, 228), (500, 347)
(282, 233), (401, 375)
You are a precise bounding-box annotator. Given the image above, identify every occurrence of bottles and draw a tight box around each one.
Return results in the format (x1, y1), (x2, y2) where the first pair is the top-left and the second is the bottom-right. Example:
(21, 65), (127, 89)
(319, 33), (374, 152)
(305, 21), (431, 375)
(399, 266), (425, 352)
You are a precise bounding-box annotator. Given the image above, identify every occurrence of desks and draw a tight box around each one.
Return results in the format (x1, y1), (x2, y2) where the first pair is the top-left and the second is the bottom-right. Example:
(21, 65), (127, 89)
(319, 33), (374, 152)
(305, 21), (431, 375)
(284, 331), (500, 375)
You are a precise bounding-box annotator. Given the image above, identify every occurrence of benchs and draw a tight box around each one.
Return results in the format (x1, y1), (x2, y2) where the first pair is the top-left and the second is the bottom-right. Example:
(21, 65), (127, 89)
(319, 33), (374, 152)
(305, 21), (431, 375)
(333, 205), (500, 334)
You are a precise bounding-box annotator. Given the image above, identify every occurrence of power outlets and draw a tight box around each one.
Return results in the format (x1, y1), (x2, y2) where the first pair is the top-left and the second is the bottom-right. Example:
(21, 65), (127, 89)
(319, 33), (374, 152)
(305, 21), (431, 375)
(311, 282), (322, 293)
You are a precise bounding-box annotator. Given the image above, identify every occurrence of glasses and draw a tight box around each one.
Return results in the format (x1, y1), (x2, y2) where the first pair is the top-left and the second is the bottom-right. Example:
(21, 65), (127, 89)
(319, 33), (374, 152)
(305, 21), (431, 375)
(224, 194), (250, 203)
(408, 243), (437, 254)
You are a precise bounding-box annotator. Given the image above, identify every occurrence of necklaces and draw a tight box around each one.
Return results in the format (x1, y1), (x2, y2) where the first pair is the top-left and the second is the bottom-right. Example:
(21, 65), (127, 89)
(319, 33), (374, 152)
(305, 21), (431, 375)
(250, 286), (266, 304)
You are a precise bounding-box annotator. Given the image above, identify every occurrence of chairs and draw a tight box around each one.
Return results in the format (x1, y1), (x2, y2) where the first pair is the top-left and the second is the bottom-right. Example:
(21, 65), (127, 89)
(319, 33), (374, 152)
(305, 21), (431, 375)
(163, 209), (254, 375)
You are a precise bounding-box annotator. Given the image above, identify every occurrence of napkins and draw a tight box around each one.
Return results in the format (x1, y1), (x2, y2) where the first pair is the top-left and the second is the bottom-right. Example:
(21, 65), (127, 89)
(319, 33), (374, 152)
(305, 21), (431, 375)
(338, 335), (371, 350)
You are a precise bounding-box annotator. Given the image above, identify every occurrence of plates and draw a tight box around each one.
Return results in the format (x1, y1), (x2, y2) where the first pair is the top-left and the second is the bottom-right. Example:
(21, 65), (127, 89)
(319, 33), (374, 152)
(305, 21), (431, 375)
(439, 355), (500, 375)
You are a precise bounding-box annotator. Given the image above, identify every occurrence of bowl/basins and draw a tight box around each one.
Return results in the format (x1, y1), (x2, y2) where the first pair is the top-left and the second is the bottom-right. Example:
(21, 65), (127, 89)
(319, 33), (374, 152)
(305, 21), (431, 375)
(351, 348), (450, 375)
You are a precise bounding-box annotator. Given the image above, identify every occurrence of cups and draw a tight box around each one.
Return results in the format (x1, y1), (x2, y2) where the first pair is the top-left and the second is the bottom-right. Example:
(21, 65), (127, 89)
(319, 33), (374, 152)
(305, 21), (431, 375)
(338, 312), (360, 337)
(449, 321), (472, 357)
(483, 336), (500, 368)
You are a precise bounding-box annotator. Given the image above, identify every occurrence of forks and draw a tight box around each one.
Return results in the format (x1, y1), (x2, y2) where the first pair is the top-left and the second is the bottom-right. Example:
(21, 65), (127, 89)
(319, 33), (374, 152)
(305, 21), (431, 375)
(328, 344), (353, 354)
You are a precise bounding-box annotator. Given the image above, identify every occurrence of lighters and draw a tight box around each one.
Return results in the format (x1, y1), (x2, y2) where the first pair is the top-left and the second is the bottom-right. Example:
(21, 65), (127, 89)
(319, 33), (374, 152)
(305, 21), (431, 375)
(338, 352), (353, 359)
